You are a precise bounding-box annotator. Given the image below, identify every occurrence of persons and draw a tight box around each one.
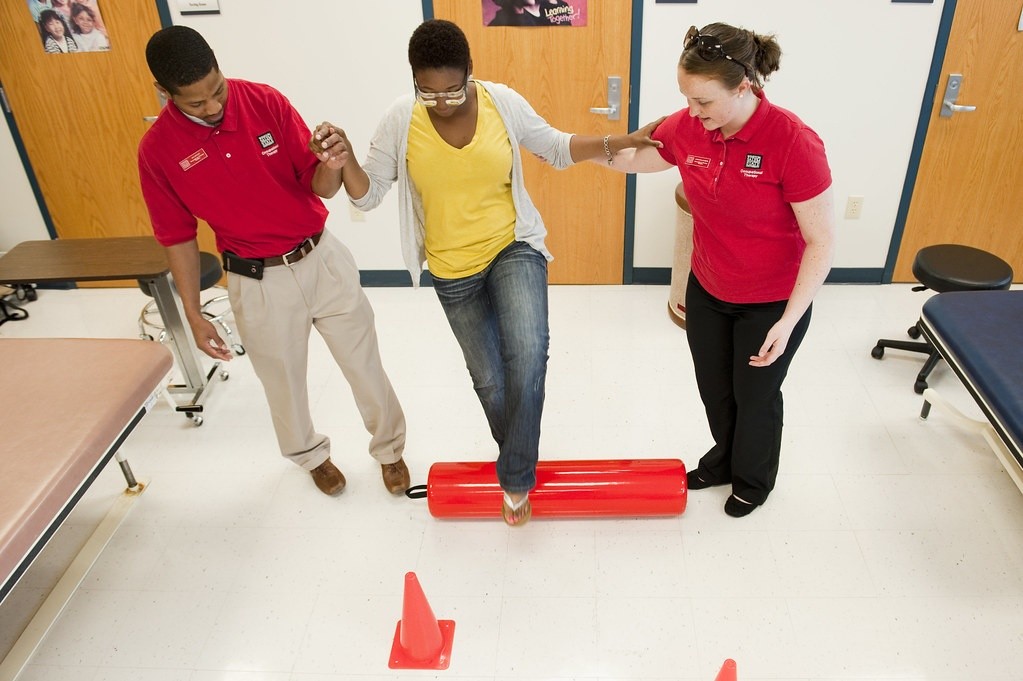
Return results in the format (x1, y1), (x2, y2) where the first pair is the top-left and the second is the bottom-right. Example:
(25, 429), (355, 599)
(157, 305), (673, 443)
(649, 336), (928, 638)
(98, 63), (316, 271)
(532, 22), (836, 518)
(487, 0), (571, 26)
(310, 20), (667, 528)
(27, 0), (108, 53)
(137, 24), (411, 495)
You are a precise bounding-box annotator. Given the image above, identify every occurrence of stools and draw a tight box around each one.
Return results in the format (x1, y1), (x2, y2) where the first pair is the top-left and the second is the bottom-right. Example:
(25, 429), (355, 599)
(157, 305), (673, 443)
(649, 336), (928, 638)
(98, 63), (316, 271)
(870, 243), (1014, 395)
(136, 252), (245, 382)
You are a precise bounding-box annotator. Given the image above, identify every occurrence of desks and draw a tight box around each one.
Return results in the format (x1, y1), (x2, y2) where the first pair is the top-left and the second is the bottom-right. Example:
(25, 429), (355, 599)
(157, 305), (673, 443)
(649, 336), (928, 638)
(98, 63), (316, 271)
(0, 235), (228, 427)
(1, 336), (174, 681)
(917, 289), (1023, 497)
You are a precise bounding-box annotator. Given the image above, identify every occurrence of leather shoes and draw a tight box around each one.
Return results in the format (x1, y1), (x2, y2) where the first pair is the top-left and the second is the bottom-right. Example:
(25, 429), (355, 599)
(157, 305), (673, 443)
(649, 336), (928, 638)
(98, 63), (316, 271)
(381, 457), (410, 496)
(310, 460), (346, 496)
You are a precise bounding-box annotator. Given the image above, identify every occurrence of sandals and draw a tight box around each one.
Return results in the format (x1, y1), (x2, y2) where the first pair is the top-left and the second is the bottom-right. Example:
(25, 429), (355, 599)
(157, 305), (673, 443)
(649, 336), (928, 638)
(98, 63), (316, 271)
(502, 490), (531, 526)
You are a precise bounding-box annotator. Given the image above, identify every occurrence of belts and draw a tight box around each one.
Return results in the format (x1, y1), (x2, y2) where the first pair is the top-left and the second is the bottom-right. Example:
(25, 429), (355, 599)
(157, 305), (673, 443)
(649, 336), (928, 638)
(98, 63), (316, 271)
(257, 232), (322, 267)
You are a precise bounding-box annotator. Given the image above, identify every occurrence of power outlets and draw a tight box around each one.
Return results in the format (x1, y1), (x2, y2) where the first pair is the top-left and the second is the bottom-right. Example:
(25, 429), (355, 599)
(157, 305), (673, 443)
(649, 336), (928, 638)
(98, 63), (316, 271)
(844, 195), (864, 219)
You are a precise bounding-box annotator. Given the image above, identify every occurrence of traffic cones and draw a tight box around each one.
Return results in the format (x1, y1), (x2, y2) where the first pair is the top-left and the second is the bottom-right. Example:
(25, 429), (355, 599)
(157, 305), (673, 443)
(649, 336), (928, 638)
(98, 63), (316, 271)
(388, 572), (456, 669)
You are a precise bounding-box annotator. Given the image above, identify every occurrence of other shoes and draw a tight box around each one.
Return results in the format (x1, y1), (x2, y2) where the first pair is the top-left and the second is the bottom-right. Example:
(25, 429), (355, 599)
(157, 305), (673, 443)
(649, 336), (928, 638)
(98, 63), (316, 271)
(724, 494), (759, 518)
(686, 469), (711, 490)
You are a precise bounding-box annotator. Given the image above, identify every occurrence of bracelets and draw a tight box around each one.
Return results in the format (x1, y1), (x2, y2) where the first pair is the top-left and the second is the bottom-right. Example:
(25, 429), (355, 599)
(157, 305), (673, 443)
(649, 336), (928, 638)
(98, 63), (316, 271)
(604, 135), (613, 165)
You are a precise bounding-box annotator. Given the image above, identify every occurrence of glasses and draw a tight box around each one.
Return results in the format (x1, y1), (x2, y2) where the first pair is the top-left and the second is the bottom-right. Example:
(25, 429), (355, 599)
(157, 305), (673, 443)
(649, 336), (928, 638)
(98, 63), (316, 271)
(682, 26), (748, 77)
(413, 62), (467, 106)
